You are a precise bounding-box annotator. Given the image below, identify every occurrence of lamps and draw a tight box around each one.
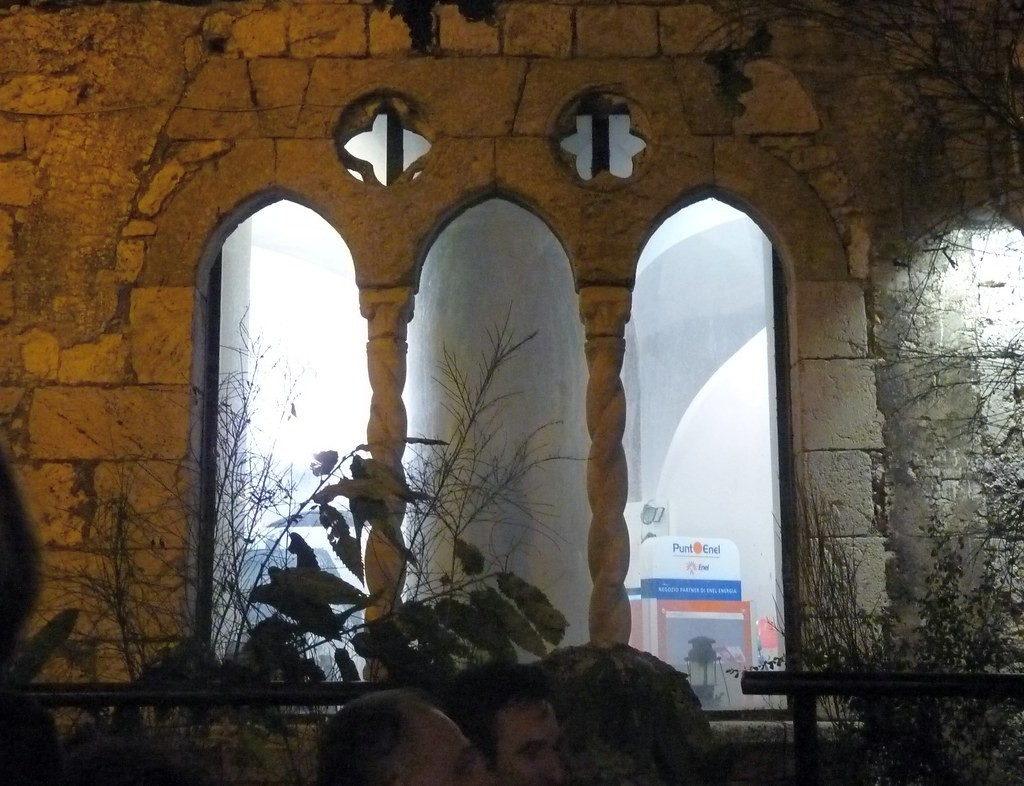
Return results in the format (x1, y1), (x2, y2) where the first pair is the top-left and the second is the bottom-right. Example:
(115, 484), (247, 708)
(684, 636), (722, 700)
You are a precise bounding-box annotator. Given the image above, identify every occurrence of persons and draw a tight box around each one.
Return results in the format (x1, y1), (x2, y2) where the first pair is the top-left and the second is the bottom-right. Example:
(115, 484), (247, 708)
(438, 664), (566, 786)
(317, 697), (498, 786)
